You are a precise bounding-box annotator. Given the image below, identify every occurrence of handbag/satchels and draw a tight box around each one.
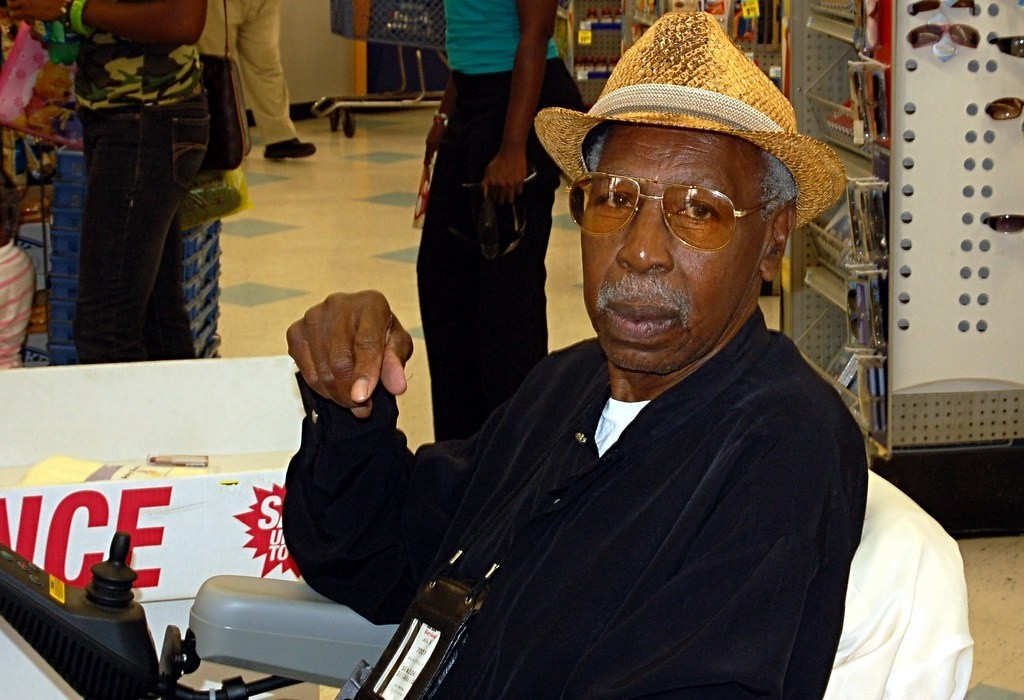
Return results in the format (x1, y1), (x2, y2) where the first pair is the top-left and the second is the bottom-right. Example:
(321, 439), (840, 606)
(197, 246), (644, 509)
(0, 20), (84, 151)
(175, 167), (251, 231)
(197, 51), (252, 171)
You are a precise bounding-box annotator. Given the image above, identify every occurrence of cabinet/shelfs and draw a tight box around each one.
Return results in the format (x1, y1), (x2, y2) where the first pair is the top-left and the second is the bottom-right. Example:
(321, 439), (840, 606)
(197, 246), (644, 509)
(551, 0), (1024, 537)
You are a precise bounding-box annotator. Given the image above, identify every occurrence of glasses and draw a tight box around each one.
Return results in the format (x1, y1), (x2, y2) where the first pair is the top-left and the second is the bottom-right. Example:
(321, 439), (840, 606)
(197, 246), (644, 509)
(414, 165), (431, 220)
(908, 23), (980, 48)
(842, 0), (891, 363)
(986, 97), (1024, 120)
(996, 36), (1024, 59)
(982, 214), (1024, 235)
(908, 0), (977, 17)
(480, 169), (538, 260)
(567, 171), (779, 250)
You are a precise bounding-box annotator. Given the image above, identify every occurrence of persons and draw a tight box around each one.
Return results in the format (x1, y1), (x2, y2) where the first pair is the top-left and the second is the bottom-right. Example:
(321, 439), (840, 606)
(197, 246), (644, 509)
(0, 0), (316, 361)
(284, 13), (866, 700)
(415, 0), (585, 449)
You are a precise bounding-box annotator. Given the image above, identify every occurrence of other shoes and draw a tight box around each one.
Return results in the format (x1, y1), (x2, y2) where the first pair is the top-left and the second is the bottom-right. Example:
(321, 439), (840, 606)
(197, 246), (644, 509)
(265, 138), (315, 158)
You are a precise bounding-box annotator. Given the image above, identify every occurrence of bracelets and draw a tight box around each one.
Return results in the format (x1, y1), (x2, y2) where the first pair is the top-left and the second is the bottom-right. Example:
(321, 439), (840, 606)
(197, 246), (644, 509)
(57, 0), (77, 37)
(433, 109), (449, 126)
(70, 0), (95, 38)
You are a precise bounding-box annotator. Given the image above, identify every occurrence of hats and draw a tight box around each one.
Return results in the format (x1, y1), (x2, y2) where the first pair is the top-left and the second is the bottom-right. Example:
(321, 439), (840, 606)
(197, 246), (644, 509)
(533, 12), (846, 228)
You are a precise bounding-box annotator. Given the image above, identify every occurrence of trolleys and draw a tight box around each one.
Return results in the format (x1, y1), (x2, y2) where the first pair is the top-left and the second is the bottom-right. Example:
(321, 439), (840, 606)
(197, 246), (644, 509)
(309, 0), (452, 137)
